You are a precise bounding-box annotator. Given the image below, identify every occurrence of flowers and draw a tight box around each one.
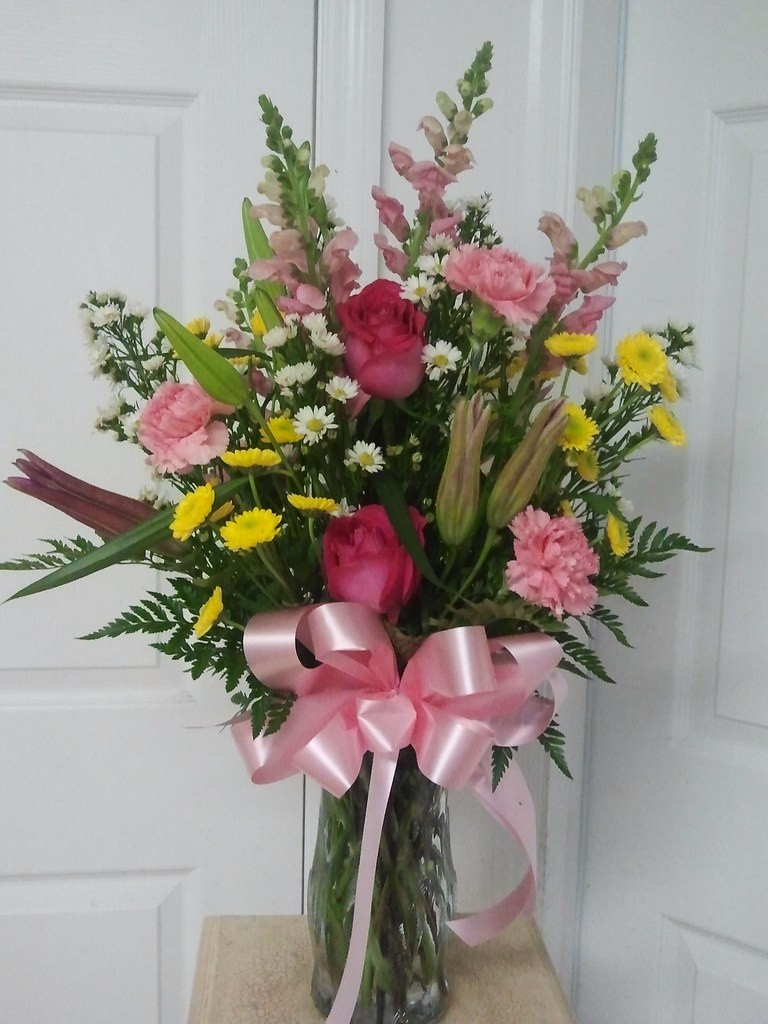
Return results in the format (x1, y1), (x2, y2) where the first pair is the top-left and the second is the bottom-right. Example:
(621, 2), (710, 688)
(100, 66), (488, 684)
(0, 38), (720, 765)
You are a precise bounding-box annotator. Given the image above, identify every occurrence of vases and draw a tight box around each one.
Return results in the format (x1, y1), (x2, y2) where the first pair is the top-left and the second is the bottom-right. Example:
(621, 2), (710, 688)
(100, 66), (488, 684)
(301, 717), (459, 1024)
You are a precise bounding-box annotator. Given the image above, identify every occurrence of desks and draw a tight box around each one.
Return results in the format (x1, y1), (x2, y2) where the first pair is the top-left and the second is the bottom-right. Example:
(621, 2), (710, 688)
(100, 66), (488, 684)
(184, 906), (576, 1023)
